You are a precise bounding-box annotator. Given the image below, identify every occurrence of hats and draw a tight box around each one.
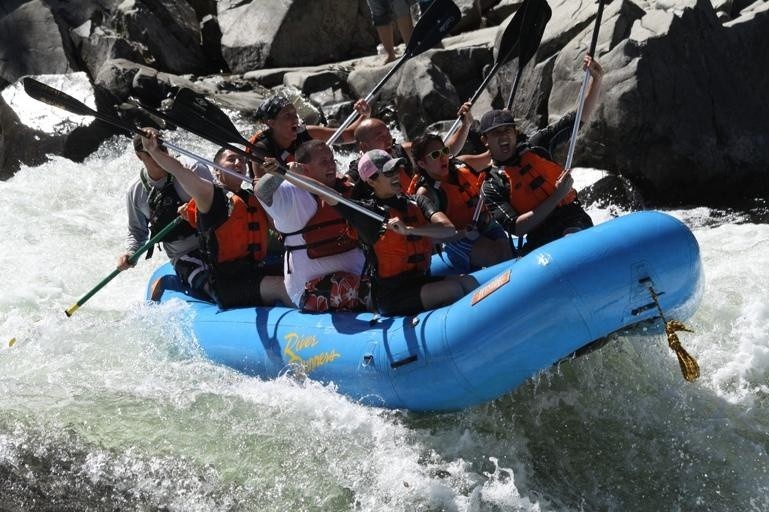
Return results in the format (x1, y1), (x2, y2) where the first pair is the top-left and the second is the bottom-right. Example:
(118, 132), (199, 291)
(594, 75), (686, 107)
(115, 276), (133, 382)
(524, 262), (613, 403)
(254, 95), (291, 122)
(478, 110), (517, 134)
(358, 149), (408, 182)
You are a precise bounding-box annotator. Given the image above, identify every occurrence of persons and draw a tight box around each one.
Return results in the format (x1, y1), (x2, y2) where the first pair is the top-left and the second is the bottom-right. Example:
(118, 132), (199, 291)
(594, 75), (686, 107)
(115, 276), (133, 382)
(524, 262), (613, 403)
(344, 102), (472, 198)
(245, 92), (370, 184)
(116, 133), (217, 304)
(259, 149), (481, 318)
(254, 140), (374, 312)
(481, 110), (594, 255)
(412, 134), (516, 268)
(141, 128), (296, 308)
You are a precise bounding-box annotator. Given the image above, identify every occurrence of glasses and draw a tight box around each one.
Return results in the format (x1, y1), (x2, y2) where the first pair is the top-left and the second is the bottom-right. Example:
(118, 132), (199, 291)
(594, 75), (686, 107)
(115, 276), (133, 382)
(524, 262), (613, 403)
(423, 147), (450, 161)
(383, 164), (402, 178)
(140, 146), (169, 156)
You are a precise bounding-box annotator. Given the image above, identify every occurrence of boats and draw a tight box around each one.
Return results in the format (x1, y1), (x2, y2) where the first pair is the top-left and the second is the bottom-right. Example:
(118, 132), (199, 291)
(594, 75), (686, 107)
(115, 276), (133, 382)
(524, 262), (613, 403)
(146, 211), (699, 409)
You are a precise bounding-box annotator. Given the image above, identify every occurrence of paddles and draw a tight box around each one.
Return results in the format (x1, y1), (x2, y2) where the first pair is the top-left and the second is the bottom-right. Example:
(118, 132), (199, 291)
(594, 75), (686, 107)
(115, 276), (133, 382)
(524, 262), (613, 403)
(442, 0), (549, 142)
(126, 95), (401, 230)
(24, 77), (256, 183)
(326, 0), (462, 145)
(169, 86), (292, 167)
(472, 3), (553, 229)
(8, 214), (187, 349)
